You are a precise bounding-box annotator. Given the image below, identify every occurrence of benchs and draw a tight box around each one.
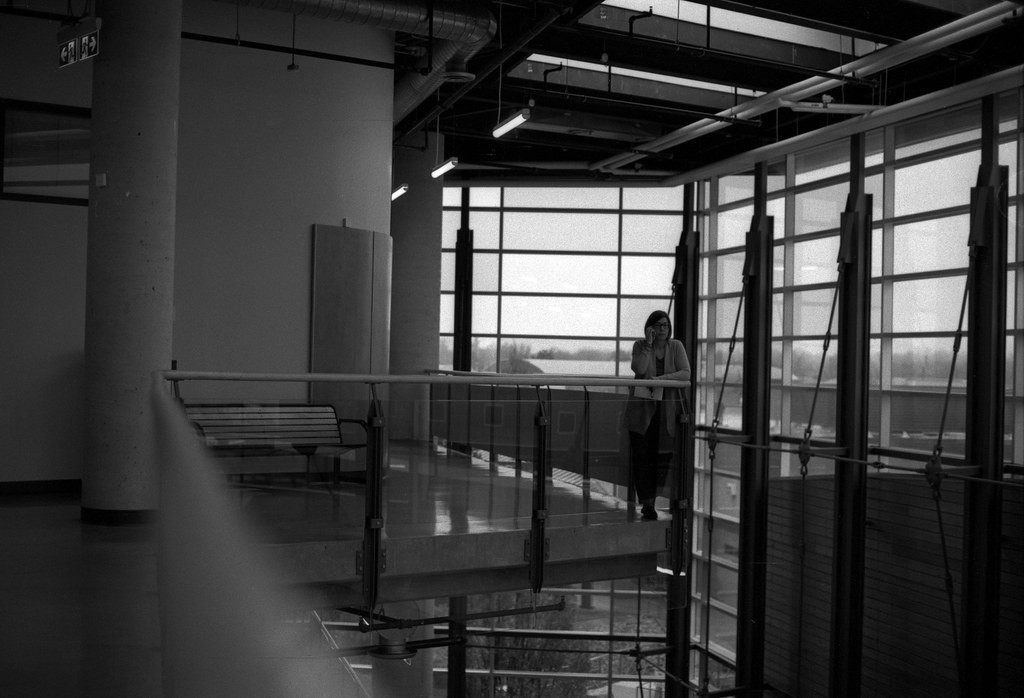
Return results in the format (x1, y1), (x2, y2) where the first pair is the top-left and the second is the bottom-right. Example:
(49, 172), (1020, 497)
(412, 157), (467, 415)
(172, 360), (368, 490)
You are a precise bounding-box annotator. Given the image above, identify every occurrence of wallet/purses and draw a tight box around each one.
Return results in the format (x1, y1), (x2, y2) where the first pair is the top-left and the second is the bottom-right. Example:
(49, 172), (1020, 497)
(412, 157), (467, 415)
(634, 386), (664, 401)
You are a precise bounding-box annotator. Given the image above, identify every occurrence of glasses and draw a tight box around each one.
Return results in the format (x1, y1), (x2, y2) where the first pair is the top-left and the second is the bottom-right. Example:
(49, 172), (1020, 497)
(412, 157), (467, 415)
(653, 322), (669, 329)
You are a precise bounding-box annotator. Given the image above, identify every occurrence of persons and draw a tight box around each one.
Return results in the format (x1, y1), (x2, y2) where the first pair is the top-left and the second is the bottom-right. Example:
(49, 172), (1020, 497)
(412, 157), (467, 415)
(622, 310), (690, 519)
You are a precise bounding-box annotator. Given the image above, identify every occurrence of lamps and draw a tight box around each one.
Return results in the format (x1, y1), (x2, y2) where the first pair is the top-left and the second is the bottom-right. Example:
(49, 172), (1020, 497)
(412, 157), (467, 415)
(431, 87), (458, 178)
(492, 0), (530, 138)
(391, 142), (408, 201)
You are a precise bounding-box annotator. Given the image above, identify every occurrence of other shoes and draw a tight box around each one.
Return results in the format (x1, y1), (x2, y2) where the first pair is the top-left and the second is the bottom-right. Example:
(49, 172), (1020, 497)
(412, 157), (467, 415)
(643, 505), (657, 521)
